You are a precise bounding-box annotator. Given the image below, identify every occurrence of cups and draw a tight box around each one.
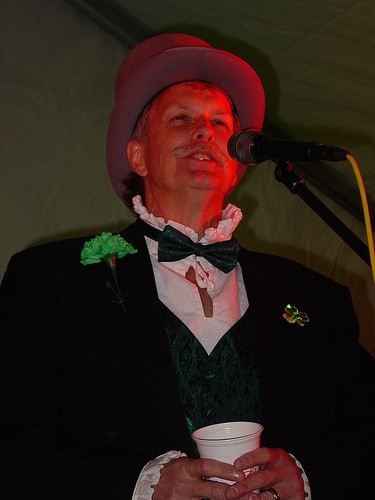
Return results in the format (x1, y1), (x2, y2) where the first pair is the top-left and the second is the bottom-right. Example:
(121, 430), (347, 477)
(191, 420), (264, 494)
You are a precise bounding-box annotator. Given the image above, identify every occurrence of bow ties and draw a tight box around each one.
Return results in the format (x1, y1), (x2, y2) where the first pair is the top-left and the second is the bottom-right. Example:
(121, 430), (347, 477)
(156, 224), (242, 273)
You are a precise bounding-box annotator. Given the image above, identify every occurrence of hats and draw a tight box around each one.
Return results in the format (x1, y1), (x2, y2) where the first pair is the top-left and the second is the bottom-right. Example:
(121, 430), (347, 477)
(106, 34), (267, 199)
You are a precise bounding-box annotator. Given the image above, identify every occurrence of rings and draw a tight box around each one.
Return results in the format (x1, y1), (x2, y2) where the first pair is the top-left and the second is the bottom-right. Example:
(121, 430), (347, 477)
(268, 487), (280, 500)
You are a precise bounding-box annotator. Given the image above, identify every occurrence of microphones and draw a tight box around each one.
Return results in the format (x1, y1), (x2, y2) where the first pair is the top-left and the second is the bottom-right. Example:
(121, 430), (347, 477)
(227, 126), (352, 166)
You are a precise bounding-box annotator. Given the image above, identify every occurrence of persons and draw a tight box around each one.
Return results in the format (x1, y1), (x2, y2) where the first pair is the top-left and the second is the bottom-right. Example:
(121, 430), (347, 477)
(0, 33), (375, 500)
